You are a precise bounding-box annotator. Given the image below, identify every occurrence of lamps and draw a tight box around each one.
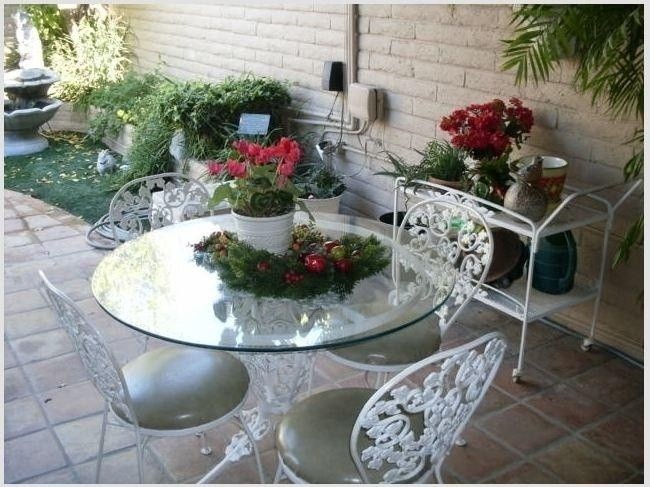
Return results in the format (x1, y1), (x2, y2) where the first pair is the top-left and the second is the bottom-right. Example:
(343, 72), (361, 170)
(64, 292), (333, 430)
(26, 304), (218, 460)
(290, 201), (491, 388)
(109, 172), (213, 248)
(37, 269), (273, 484)
(270, 331), (508, 484)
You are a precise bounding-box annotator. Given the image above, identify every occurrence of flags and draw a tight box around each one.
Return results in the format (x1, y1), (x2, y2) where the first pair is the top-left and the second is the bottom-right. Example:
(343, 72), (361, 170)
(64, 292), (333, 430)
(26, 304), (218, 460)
(519, 154), (568, 212)
(226, 209), (297, 260)
(481, 177), (517, 213)
(379, 210), (427, 283)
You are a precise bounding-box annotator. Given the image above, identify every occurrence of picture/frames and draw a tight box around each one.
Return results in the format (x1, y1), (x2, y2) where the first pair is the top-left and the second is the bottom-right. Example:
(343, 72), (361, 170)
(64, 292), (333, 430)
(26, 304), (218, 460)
(91, 213), (458, 485)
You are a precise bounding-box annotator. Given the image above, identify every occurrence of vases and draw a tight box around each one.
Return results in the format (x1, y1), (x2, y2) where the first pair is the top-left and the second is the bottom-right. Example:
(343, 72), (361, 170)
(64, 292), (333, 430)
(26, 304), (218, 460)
(439, 96), (535, 197)
(204, 135), (306, 213)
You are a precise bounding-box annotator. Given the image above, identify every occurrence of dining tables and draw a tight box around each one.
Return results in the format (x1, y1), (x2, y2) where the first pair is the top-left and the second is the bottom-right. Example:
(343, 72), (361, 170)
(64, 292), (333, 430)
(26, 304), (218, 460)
(291, 161), (349, 213)
(418, 136), (473, 196)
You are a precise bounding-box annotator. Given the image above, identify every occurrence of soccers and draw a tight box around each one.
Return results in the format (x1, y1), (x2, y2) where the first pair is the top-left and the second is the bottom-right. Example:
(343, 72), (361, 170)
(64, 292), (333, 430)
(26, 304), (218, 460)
(305, 255), (326, 273)
(335, 260), (351, 273)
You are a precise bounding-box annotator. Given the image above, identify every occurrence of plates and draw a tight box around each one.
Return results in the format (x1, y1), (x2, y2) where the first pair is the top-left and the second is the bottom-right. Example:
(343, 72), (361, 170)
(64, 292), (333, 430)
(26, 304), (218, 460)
(389, 166), (644, 386)
